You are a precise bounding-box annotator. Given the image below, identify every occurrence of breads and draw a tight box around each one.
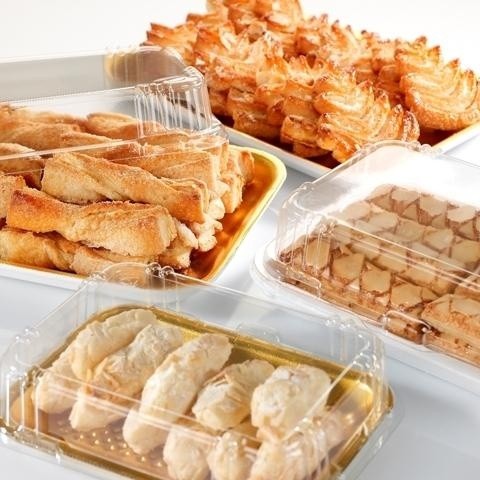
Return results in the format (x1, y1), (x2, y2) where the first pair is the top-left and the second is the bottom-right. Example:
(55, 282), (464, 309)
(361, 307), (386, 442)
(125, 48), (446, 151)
(0, 0), (480, 480)
